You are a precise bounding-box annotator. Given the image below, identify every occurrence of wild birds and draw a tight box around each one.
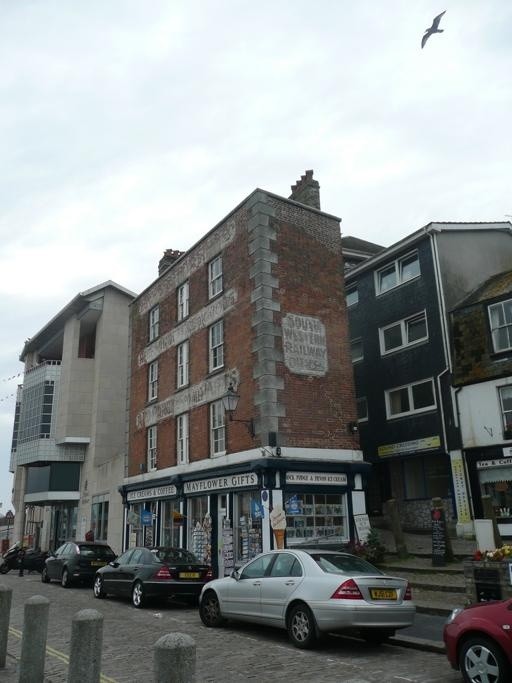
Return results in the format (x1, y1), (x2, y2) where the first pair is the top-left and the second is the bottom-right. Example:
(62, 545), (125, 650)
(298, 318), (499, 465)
(418, 7), (449, 49)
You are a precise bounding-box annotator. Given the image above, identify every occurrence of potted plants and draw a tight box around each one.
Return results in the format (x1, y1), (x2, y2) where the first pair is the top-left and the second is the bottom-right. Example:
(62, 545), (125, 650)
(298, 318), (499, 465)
(359, 527), (386, 567)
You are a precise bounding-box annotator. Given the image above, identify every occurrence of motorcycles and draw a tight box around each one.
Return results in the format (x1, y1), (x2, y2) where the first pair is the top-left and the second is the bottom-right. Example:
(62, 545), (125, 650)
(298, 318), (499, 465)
(0, 543), (50, 574)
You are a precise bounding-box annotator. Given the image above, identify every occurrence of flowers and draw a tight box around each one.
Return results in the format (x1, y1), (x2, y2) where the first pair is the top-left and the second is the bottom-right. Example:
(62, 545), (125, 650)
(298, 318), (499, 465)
(473, 543), (511, 563)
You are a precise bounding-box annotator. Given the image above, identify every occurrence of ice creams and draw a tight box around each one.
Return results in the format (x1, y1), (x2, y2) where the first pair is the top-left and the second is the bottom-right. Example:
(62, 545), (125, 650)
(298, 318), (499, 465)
(269, 505), (287, 549)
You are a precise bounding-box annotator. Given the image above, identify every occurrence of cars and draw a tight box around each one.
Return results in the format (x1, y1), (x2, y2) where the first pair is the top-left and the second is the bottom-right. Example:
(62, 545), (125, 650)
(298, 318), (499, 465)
(42, 541), (215, 609)
(443, 597), (512, 683)
(198, 549), (416, 649)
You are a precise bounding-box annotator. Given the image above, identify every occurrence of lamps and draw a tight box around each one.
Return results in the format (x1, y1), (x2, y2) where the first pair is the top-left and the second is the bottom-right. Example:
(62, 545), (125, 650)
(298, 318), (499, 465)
(219, 384), (255, 439)
